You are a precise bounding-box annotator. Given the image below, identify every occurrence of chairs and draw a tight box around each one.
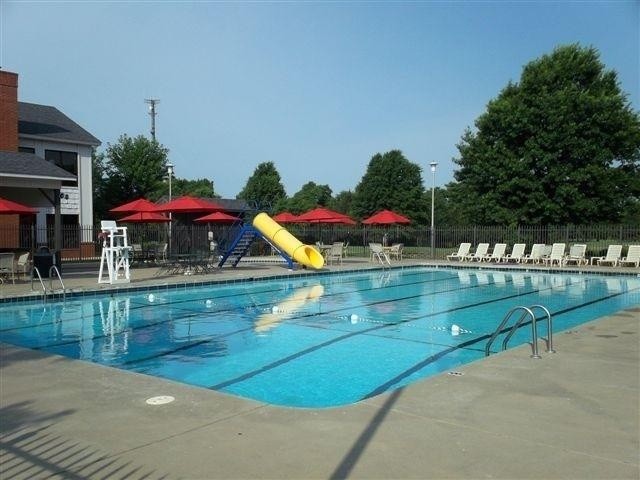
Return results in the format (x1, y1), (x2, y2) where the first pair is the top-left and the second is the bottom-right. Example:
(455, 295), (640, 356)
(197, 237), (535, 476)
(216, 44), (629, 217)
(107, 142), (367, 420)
(128, 244), (218, 275)
(447, 243), (640, 267)
(369, 243), (404, 266)
(0, 252), (30, 285)
(101, 221), (128, 247)
(313, 242), (349, 266)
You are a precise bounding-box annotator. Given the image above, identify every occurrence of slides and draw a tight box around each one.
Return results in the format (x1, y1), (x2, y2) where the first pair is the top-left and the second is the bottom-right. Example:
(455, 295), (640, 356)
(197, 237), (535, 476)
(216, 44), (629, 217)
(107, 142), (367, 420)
(252, 212), (324, 269)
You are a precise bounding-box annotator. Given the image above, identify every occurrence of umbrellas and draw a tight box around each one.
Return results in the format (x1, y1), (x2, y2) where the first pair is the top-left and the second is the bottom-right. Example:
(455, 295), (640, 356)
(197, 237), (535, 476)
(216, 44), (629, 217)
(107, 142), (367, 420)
(361, 210), (411, 247)
(273, 206), (357, 251)
(0, 197), (39, 214)
(108, 195), (242, 264)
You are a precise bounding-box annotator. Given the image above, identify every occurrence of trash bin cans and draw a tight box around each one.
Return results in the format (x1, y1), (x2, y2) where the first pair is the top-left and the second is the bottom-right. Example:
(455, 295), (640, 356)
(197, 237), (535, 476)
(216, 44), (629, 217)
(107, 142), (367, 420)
(33, 246), (61, 278)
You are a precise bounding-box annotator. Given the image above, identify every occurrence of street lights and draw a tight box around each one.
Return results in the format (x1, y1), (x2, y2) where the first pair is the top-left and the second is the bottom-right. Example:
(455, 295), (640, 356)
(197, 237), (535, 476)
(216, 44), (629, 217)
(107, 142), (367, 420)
(165, 161), (174, 257)
(428, 161), (439, 258)
